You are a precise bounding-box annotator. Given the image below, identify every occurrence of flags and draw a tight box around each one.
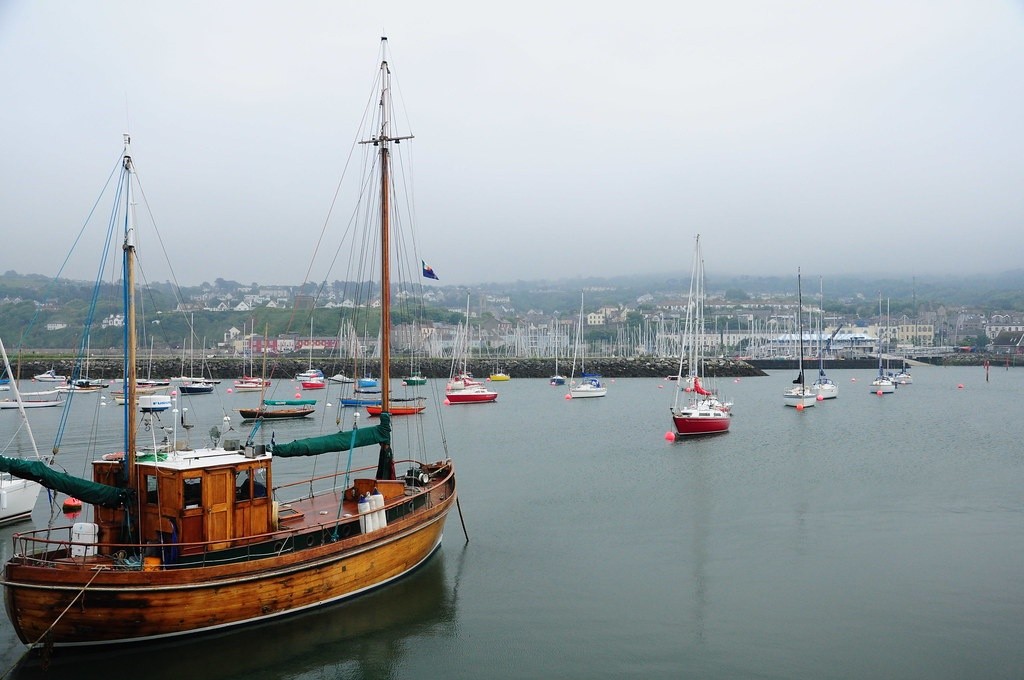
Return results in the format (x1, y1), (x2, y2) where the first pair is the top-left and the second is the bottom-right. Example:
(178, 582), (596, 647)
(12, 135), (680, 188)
(422, 259), (439, 280)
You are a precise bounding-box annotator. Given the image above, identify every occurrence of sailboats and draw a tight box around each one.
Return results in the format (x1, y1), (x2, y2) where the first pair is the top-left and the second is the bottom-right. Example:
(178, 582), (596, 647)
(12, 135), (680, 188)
(357, 324), (379, 388)
(0, 34), (460, 652)
(445, 293), (511, 404)
(670, 233), (734, 435)
(113, 312), (221, 406)
(870, 291), (912, 395)
(550, 317), (566, 386)
(296, 317), (326, 391)
(0, 326), (66, 408)
(231, 320), (315, 420)
(56, 333), (107, 394)
(568, 289), (606, 398)
(402, 319), (428, 386)
(811, 273), (838, 400)
(235, 318), (271, 392)
(782, 267), (816, 409)
(336, 345), (427, 415)
(736, 314), (846, 360)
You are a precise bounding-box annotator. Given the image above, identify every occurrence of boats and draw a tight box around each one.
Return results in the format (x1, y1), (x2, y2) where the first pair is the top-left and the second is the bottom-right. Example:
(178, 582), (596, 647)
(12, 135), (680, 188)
(327, 373), (354, 383)
(34, 370), (65, 382)
